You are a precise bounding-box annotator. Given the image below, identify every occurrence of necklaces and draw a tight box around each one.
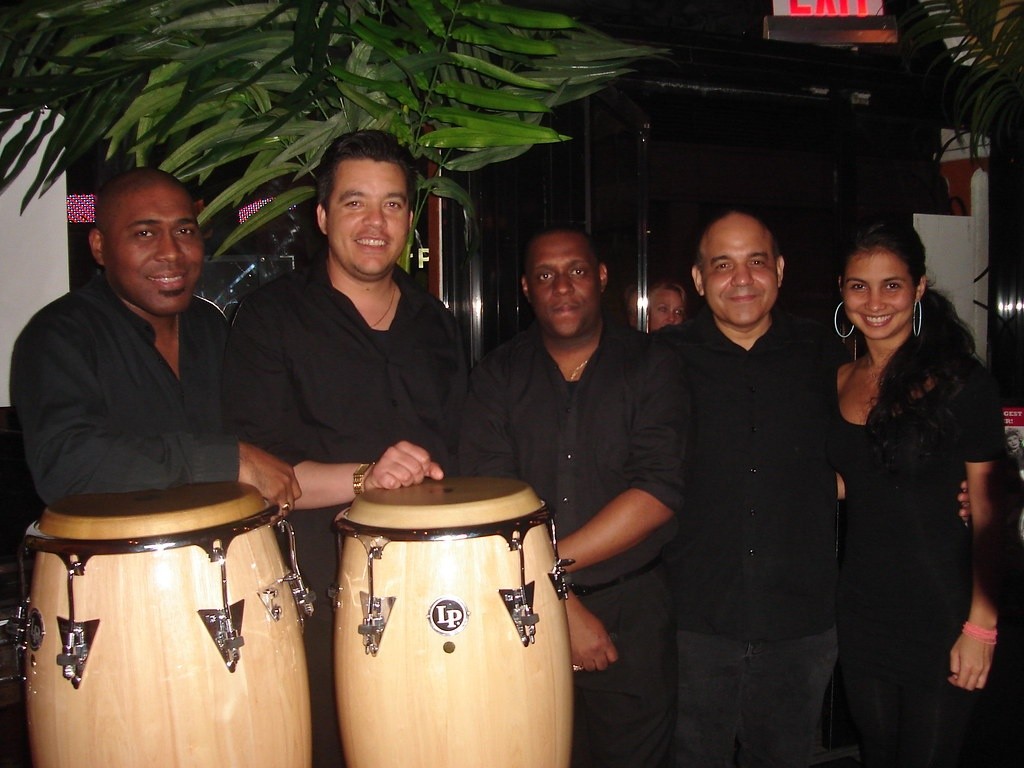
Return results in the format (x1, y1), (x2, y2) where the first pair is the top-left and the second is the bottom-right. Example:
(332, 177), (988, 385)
(370, 289), (396, 327)
(570, 361), (587, 380)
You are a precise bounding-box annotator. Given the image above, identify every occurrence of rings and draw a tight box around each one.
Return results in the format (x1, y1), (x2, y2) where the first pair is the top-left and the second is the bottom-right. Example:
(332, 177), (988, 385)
(282, 503), (290, 510)
(572, 665), (583, 671)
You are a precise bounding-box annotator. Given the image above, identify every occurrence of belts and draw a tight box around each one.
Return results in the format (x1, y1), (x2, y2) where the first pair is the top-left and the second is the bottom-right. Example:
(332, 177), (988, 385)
(568, 562), (660, 597)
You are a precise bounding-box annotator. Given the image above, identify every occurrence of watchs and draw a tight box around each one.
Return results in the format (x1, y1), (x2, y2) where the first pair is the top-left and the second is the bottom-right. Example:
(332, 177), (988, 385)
(353, 462), (373, 495)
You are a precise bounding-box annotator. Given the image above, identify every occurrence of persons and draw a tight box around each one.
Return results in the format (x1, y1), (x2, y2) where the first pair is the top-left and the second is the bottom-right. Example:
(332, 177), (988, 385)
(1005, 428), (1024, 471)
(224, 131), (471, 767)
(823, 218), (1009, 767)
(645, 210), (1024, 767)
(464, 223), (692, 767)
(8, 166), (302, 552)
(628, 274), (685, 333)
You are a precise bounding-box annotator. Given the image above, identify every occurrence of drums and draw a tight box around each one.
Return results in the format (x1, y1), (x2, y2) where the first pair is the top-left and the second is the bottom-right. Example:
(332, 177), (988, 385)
(21, 479), (317, 768)
(320, 474), (577, 768)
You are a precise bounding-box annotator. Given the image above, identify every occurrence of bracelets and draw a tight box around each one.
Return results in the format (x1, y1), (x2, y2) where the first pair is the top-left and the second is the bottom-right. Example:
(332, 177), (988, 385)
(962, 622), (998, 645)
